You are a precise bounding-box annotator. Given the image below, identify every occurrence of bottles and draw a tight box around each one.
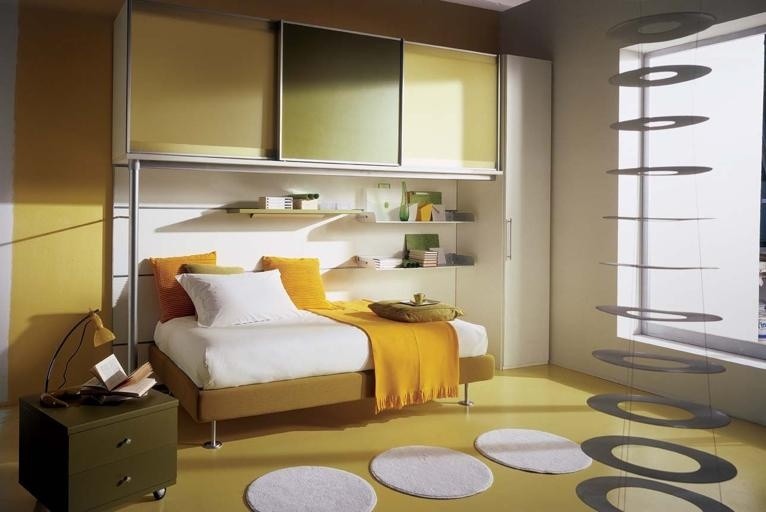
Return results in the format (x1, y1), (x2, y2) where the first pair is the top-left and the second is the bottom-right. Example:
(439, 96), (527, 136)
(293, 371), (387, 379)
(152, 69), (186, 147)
(399, 182), (409, 222)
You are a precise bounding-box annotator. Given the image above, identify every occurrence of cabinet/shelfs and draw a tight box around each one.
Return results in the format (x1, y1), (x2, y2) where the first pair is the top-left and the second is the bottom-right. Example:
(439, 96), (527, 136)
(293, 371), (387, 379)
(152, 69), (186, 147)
(19, 398), (180, 511)
(228, 208), (476, 270)
(456, 55), (553, 370)
(113, 0), (504, 180)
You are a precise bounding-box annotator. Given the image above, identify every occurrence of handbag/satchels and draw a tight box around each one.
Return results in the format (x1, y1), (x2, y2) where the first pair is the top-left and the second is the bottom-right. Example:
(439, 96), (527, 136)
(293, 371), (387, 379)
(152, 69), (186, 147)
(366, 183), (402, 221)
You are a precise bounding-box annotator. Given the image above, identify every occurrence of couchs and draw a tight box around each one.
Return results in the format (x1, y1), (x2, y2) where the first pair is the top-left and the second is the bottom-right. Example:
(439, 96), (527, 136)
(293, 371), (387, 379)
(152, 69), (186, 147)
(148, 299), (495, 448)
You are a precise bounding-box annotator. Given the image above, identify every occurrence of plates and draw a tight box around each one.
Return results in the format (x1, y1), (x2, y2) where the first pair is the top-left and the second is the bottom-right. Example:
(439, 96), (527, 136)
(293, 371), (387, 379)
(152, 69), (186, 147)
(400, 298), (441, 306)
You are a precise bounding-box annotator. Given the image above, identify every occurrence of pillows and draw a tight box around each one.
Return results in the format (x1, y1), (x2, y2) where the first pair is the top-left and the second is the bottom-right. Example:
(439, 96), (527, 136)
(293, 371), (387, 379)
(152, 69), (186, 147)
(183, 264), (244, 321)
(369, 299), (463, 322)
(175, 269), (303, 328)
(262, 256), (330, 310)
(150, 251), (217, 323)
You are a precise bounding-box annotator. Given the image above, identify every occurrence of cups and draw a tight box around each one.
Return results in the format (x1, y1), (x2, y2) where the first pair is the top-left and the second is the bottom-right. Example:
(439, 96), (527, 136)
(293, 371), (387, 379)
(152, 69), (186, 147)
(413, 292), (425, 303)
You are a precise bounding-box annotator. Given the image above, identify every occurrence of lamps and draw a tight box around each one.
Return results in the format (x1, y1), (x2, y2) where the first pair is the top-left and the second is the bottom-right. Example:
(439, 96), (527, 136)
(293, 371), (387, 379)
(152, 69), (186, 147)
(40, 308), (116, 409)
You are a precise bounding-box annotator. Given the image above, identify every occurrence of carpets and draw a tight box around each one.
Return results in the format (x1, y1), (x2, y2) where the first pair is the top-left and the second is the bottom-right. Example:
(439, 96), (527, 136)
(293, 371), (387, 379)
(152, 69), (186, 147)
(474, 428), (593, 474)
(369, 446), (494, 499)
(245, 466), (378, 512)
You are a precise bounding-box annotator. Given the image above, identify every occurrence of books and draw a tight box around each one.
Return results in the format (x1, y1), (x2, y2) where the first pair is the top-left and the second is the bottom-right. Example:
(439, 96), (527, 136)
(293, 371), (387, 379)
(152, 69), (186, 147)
(74, 352), (157, 401)
(357, 257), (402, 270)
(408, 249), (438, 267)
